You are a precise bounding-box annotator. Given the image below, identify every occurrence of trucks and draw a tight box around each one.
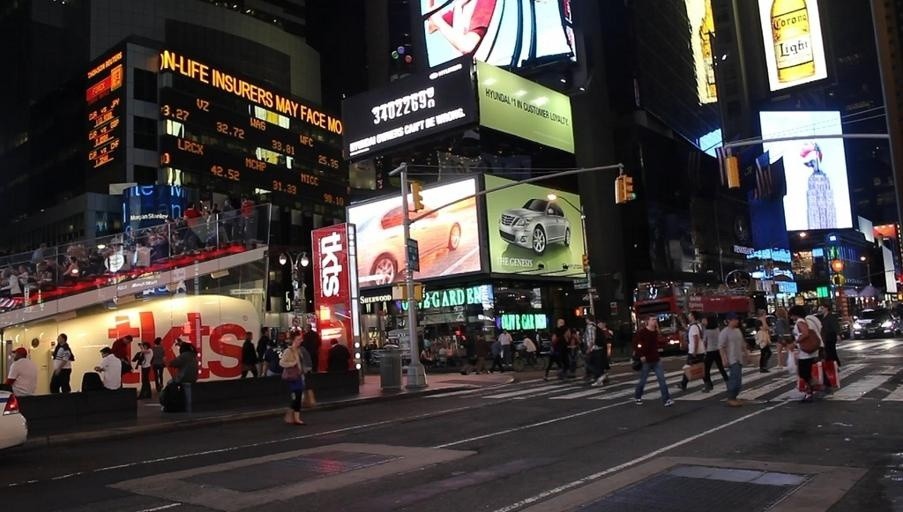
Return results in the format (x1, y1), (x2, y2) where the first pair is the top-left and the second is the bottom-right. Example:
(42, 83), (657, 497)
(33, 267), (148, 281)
(385, 328), (425, 360)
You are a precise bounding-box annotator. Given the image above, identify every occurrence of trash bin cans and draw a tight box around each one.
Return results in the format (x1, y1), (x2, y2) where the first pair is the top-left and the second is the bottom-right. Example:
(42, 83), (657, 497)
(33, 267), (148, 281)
(379, 349), (402, 393)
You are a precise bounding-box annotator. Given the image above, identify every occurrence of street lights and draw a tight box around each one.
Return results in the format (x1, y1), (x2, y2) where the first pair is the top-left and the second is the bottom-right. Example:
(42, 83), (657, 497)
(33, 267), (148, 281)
(279, 250), (309, 329)
(770, 232), (807, 310)
(860, 255), (870, 284)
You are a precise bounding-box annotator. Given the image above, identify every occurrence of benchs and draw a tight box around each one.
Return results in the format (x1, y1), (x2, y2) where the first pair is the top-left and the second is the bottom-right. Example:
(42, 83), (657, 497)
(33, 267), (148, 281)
(183, 366), (360, 410)
(17, 388), (138, 441)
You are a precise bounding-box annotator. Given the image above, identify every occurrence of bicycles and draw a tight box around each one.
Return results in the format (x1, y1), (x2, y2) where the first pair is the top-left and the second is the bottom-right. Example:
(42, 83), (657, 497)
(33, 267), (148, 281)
(511, 350), (547, 371)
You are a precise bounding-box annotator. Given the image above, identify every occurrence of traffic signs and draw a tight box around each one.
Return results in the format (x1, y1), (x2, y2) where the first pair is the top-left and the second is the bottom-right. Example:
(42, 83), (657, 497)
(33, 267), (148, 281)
(573, 279), (588, 283)
(574, 285), (589, 289)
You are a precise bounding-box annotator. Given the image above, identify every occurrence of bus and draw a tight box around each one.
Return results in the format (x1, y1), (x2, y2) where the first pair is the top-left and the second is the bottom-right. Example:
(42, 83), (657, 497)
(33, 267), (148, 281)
(630, 281), (751, 354)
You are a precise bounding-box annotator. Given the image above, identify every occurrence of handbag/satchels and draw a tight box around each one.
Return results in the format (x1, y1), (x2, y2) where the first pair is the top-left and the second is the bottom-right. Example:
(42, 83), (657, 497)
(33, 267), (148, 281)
(799, 329), (821, 354)
(755, 330), (771, 348)
(281, 363), (301, 380)
(823, 360), (840, 389)
(797, 362), (823, 392)
(683, 363), (705, 380)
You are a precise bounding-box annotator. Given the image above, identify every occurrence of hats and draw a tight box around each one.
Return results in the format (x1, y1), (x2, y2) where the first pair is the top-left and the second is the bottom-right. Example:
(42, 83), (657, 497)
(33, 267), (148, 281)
(10, 347), (27, 356)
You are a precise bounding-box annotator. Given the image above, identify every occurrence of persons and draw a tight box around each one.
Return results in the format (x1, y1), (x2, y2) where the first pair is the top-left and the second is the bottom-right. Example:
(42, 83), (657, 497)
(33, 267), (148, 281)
(238, 320), (353, 427)
(630, 310), (751, 407)
(419, 328), (544, 374)
(0, 194), (264, 309)
(542, 315), (616, 389)
(0, 331), (199, 413)
(753, 301), (842, 402)
(424, 0), (497, 56)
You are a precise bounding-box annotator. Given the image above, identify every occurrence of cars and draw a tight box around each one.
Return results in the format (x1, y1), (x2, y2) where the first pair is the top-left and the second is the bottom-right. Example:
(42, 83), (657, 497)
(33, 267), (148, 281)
(367, 349), (383, 368)
(813, 311), (851, 341)
(354, 203), (463, 285)
(498, 197), (573, 256)
(0, 390), (28, 454)
(894, 302), (903, 334)
(852, 308), (900, 338)
(743, 314), (778, 343)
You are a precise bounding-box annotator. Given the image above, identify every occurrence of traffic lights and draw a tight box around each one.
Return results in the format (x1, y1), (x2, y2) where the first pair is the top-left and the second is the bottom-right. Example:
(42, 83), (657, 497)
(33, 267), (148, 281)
(583, 256), (590, 272)
(420, 285), (425, 300)
(625, 177), (638, 202)
(417, 185), (425, 208)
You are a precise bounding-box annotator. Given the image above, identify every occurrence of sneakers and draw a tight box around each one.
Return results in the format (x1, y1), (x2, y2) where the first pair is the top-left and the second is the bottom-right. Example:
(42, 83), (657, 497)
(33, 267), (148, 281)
(663, 399), (674, 406)
(591, 374), (605, 387)
(634, 397), (642, 404)
